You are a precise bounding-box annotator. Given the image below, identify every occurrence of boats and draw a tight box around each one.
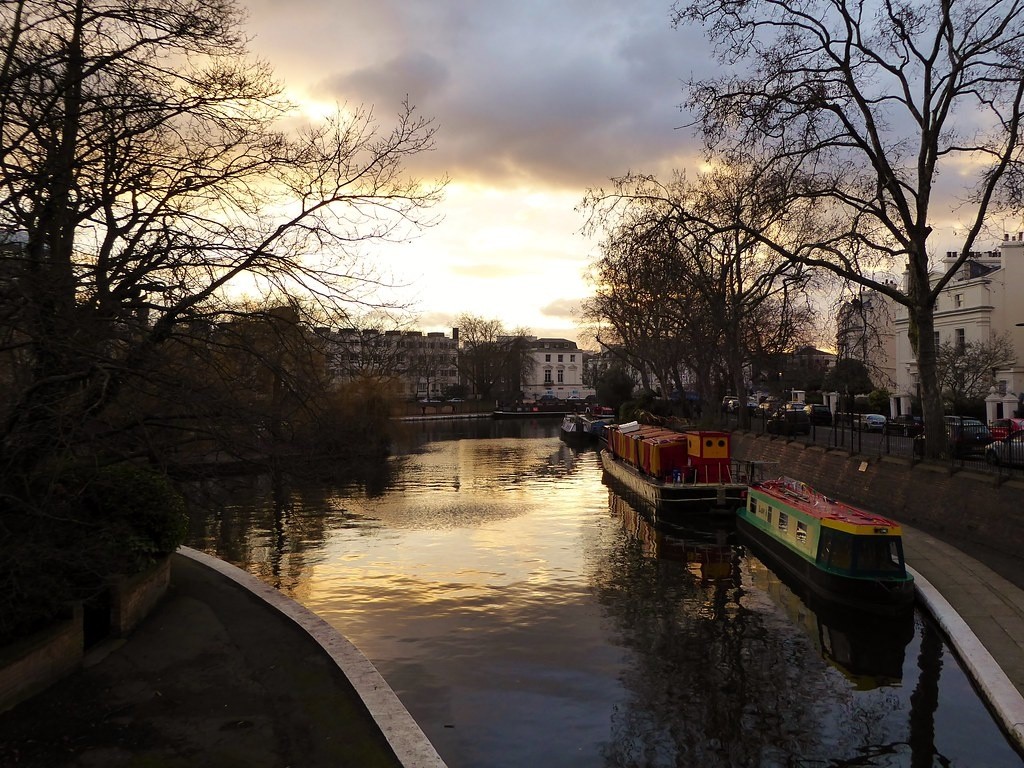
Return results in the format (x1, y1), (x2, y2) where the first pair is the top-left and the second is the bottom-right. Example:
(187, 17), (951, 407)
(601, 470), (740, 581)
(560, 414), (593, 440)
(571, 402), (615, 436)
(600, 420), (748, 517)
(733, 475), (915, 608)
(492, 399), (572, 417)
(733, 520), (916, 692)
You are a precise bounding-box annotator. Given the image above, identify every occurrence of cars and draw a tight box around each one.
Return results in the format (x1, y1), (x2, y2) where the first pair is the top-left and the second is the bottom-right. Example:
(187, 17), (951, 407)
(852, 414), (886, 431)
(418, 397), (442, 404)
(803, 404), (832, 426)
(448, 397), (467, 404)
(722, 397), (758, 413)
(753, 401), (783, 418)
(984, 429), (1024, 467)
(780, 402), (806, 410)
(881, 413), (925, 437)
(986, 417), (1024, 441)
(766, 409), (811, 436)
(922, 416), (992, 449)
